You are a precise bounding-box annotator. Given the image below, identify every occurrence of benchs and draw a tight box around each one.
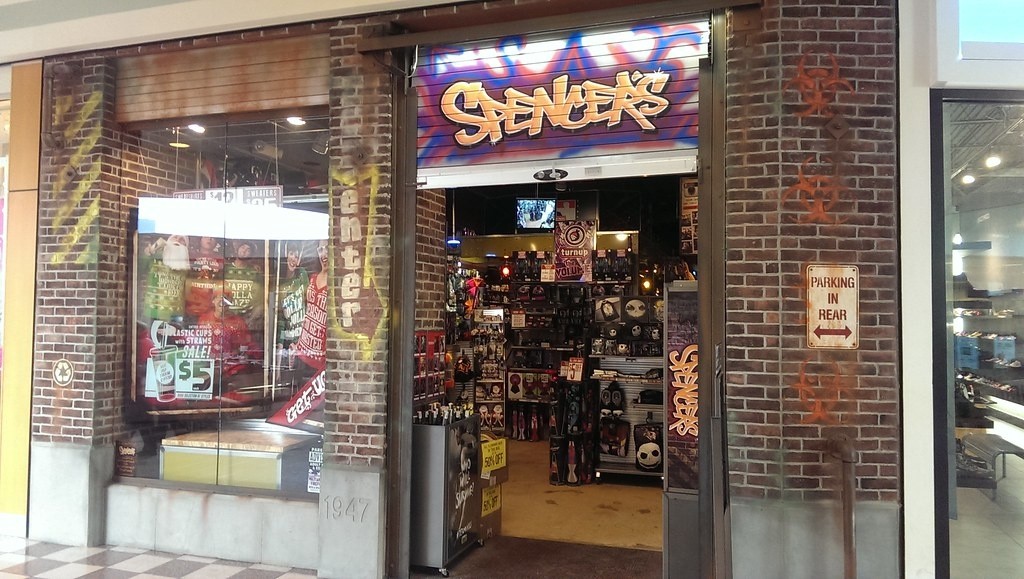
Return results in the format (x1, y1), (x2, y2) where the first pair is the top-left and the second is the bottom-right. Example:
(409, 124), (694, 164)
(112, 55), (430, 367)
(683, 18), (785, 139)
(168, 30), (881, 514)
(961, 432), (1024, 501)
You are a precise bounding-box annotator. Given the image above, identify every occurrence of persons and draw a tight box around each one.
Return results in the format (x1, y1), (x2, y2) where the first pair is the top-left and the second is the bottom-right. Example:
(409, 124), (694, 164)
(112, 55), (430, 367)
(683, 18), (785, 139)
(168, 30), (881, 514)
(275, 242), (309, 382)
(453, 425), (478, 471)
(139, 235), (263, 330)
(289, 238), (328, 390)
(191, 279), (262, 379)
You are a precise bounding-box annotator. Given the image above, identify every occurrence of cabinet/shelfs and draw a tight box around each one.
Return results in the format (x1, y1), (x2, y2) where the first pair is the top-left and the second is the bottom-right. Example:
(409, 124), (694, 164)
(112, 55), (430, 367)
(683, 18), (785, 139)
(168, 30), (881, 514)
(408, 247), (698, 573)
(954, 267), (1024, 489)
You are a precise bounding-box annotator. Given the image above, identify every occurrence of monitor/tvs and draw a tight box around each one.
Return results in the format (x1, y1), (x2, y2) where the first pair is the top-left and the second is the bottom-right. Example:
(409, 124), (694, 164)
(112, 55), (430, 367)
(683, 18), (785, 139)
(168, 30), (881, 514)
(515, 197), (555, 231)
